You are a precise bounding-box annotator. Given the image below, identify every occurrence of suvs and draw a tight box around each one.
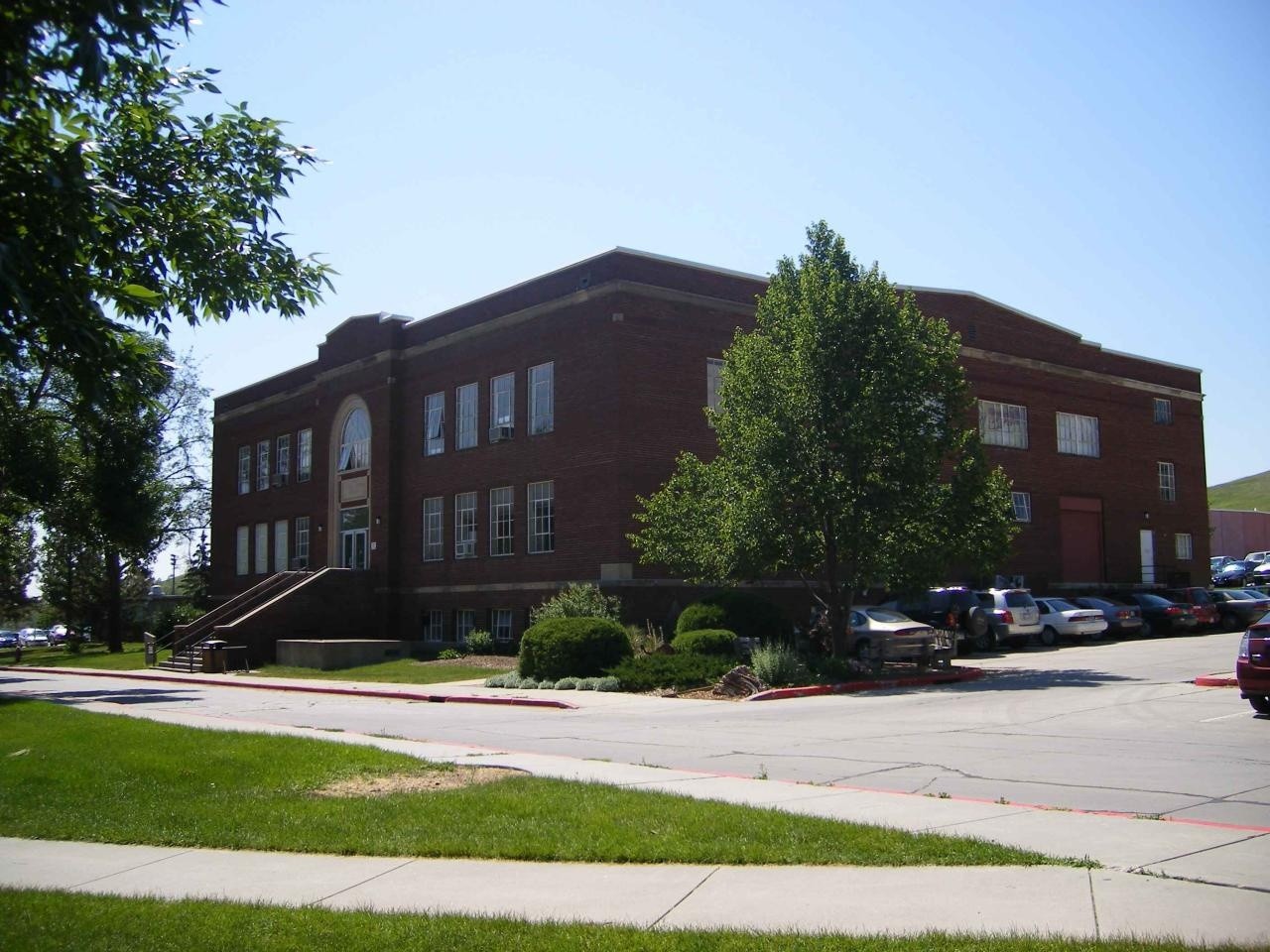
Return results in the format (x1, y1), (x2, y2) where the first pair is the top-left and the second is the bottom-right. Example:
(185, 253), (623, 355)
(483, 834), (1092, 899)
(1155, 587), (1222, 634)
(877, 586), (989, 657)
(976, 588), (1045, 651)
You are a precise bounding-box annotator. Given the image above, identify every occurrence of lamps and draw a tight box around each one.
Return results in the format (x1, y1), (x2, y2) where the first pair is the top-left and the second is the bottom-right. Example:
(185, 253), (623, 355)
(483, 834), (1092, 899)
(317, 524), (323, 533)
(376, 516), (381, 524)
(1144, 510), (1150, 519)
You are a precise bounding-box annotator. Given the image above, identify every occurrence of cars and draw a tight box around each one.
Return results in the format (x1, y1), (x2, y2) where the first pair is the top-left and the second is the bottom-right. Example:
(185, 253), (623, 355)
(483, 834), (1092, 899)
(0, 630), (17, 648)
(1071, 596), (1143, 642)
(1210, 551), (1270, 635)
(19, 628), (48, 648)
(843, 604), (935, 668)
(1237, 610), (1270, 714)
(1033, 596), (1109, 647)
(48, 624), (75, 647)
(1102, 593), (1199, 640)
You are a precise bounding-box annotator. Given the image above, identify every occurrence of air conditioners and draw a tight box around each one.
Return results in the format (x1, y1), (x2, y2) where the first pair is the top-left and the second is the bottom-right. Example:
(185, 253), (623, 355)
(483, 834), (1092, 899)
(272, 473), (287, 485)
(489, 427), (515, 440)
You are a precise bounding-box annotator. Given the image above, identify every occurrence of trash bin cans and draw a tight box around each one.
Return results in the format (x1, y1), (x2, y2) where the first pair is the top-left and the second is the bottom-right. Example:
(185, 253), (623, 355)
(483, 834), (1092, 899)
(201, 640), (228, 672)
